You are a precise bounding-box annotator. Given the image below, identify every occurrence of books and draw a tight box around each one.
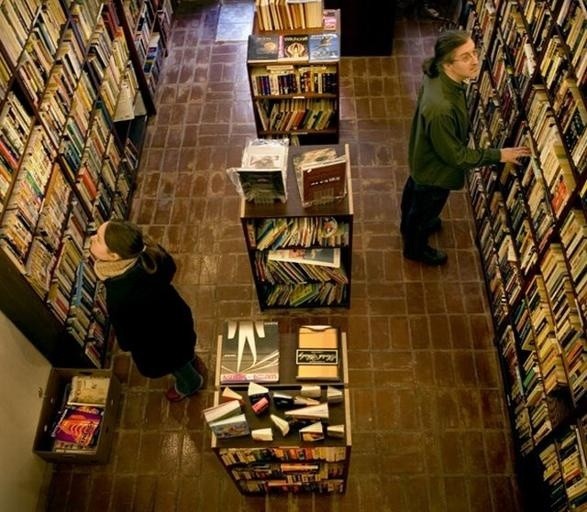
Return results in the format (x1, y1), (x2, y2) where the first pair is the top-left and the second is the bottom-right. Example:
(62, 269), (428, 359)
(0, 0), (173, 460)
(200, 316), (346, 496)
(450, 0), (587, 512)
(232, 1), (352, 309)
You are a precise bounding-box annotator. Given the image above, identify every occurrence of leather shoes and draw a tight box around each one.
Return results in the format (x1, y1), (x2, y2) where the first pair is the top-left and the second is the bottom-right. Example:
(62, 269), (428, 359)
(402, 242), (447, 266)
(425, 216), (442, 236)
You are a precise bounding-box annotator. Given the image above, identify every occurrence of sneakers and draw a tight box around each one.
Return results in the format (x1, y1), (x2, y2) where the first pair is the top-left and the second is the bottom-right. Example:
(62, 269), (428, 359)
(190, 353), (208, 376)
(165, 374), (204, 402)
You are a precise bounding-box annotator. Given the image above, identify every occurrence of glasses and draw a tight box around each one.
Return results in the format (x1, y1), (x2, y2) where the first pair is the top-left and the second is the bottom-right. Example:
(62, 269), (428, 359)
(451, 49), (477, 63)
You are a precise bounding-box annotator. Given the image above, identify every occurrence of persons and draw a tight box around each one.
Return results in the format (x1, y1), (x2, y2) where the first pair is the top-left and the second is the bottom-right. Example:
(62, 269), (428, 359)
(400, 29), (531, 266)
(88, 219), (206, 404)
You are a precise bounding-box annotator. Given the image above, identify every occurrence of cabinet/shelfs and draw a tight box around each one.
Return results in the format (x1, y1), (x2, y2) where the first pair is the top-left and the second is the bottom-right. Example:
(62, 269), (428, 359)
(213, 331), (353, 497)
(235, 142), (353, 314)
(245, 32), (345, 146)
(448, 1), (587, 512)
(1, 1), (176, 369)
